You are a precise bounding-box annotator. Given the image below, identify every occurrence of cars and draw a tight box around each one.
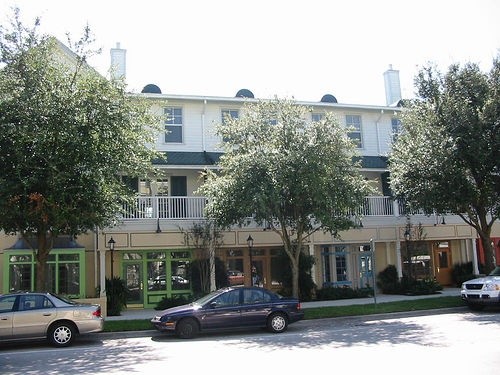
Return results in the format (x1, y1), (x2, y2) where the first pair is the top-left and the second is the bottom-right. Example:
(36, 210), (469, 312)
(0, 293), (105, 348)
(150, 284), (305, 339)
(461, 266), (500, 311)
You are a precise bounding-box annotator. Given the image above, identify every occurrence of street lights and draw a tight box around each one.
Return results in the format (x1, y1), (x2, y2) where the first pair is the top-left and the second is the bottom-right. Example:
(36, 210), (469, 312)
(107, 237), (115, 280)
(246, 235), (255, 285)
(404, 231), (412, 283)
